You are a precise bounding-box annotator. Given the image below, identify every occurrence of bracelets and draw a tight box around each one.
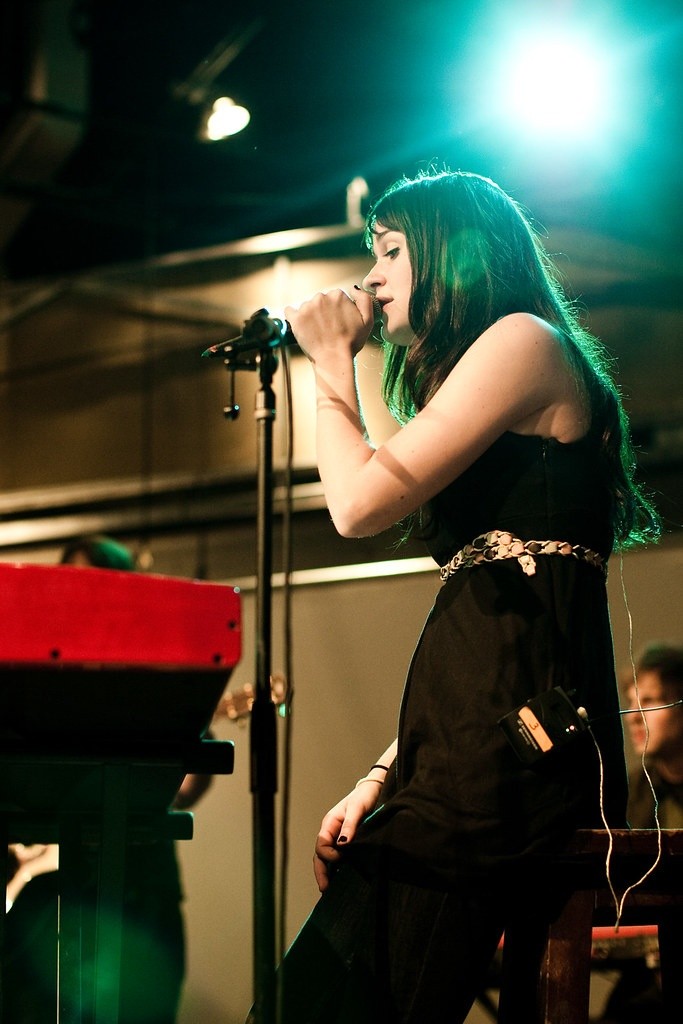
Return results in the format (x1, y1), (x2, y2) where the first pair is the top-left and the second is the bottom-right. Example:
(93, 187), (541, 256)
(355, 778), (384, 786)
(370, 765), (388, 772)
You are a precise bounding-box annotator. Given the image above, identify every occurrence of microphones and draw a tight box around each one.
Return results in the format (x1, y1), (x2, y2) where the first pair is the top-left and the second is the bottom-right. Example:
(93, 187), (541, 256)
(201, 296), (382, 360)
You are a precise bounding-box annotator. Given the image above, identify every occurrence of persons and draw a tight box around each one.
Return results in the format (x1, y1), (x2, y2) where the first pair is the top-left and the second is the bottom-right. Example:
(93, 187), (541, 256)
(56, 535), (218, 1024)
(588, 639), (683, 1024)
(246, 171), (663, 1024)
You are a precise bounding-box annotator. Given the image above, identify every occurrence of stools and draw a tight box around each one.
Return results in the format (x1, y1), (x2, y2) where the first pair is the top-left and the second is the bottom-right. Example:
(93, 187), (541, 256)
(494, 823), (683, 1024)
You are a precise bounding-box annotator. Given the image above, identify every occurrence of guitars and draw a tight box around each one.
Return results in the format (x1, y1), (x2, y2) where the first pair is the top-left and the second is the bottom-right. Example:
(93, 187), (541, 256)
(214, 675), (294, 728)
